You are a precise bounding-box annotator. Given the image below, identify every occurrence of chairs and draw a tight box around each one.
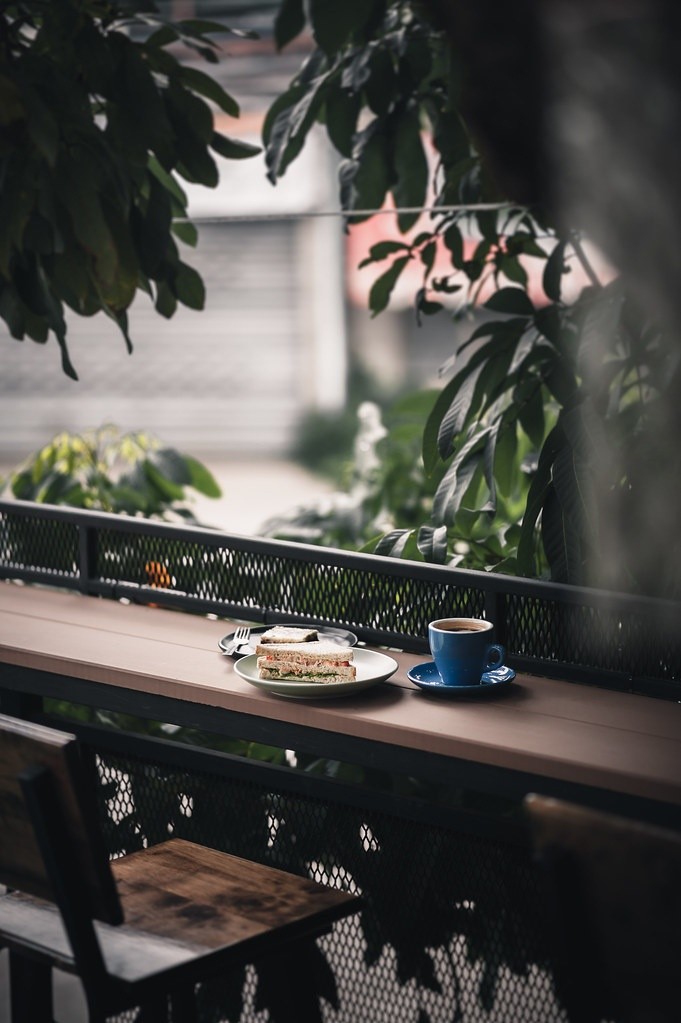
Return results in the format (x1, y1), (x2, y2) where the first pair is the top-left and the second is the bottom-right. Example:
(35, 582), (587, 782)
(0, 713), (363, 1023)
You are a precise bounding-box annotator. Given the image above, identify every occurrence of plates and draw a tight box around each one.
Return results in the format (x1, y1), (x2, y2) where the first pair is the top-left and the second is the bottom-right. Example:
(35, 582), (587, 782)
(218, 624), (358, 659)
(408, 661), (516, 694)
(234, 647), (398, 699)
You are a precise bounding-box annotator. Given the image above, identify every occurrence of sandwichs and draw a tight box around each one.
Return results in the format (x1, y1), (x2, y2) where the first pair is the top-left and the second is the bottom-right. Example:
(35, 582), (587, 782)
(256, 639), (356, 685)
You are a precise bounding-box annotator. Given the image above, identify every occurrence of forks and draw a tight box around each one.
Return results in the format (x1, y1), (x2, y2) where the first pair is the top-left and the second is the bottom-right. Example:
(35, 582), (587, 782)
(223, 627), (251, 656)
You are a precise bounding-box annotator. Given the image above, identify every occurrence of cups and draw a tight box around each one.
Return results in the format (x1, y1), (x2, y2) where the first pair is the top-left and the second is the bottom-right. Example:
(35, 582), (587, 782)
(428, 618), (507, 686)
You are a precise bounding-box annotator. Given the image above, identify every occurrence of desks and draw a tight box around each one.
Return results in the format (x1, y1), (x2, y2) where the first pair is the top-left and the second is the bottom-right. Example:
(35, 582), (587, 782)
(0, 576), (681, 881)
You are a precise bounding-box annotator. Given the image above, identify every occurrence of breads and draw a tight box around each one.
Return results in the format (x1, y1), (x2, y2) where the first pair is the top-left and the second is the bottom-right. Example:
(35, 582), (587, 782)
(260, 625), (319, 644)
